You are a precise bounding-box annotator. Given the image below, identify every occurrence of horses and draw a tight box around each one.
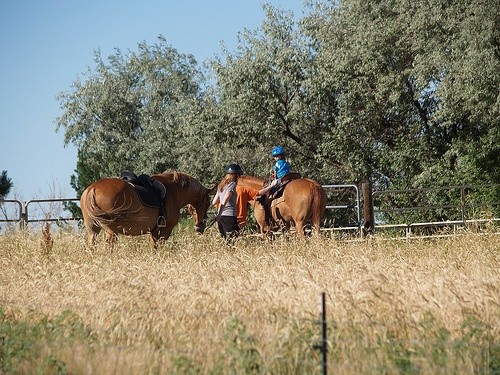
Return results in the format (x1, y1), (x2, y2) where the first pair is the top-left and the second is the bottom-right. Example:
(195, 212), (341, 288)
(235, 175), (327, 239)
(80, 171), (217, 256)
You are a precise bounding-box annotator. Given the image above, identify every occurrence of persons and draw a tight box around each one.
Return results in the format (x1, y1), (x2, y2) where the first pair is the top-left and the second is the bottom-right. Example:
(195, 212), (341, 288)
(214, 180), (279, 231)
(256, 147), (294, 207)
(212, 164), (244, 246)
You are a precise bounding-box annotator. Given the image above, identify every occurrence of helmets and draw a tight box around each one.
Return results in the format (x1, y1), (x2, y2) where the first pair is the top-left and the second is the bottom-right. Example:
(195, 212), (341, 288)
(272, 146), (284, 156)
(227, 164), (243, 175)
